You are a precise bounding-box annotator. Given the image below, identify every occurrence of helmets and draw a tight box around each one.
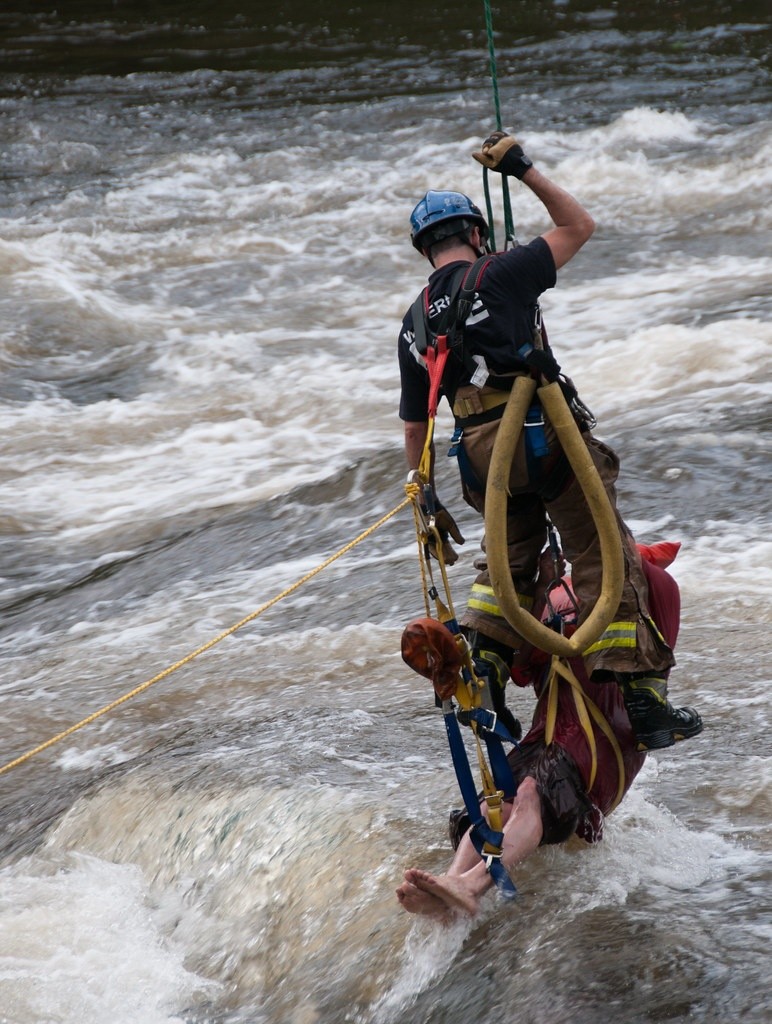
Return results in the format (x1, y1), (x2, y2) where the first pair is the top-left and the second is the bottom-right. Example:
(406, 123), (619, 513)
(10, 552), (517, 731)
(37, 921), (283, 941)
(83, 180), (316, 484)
(409, 189), (490, 243)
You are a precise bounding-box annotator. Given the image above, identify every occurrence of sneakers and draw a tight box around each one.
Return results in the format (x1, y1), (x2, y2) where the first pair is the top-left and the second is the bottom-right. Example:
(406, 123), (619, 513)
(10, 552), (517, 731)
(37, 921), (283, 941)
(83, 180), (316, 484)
(622, 688), (702, 750)
(457, 687), (522, 741)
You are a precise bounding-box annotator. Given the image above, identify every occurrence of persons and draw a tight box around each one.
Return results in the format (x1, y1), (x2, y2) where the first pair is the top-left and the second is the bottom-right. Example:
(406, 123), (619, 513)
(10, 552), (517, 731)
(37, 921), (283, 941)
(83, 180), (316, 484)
(396, 539), (682, 925)
(395, 127), (705, 750)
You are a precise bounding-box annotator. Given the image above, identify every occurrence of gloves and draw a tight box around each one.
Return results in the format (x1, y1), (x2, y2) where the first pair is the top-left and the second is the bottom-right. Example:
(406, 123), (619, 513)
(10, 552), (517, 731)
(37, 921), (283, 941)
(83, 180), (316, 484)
(420, 498), (465, 566)
(473, 131), (533, 180)
